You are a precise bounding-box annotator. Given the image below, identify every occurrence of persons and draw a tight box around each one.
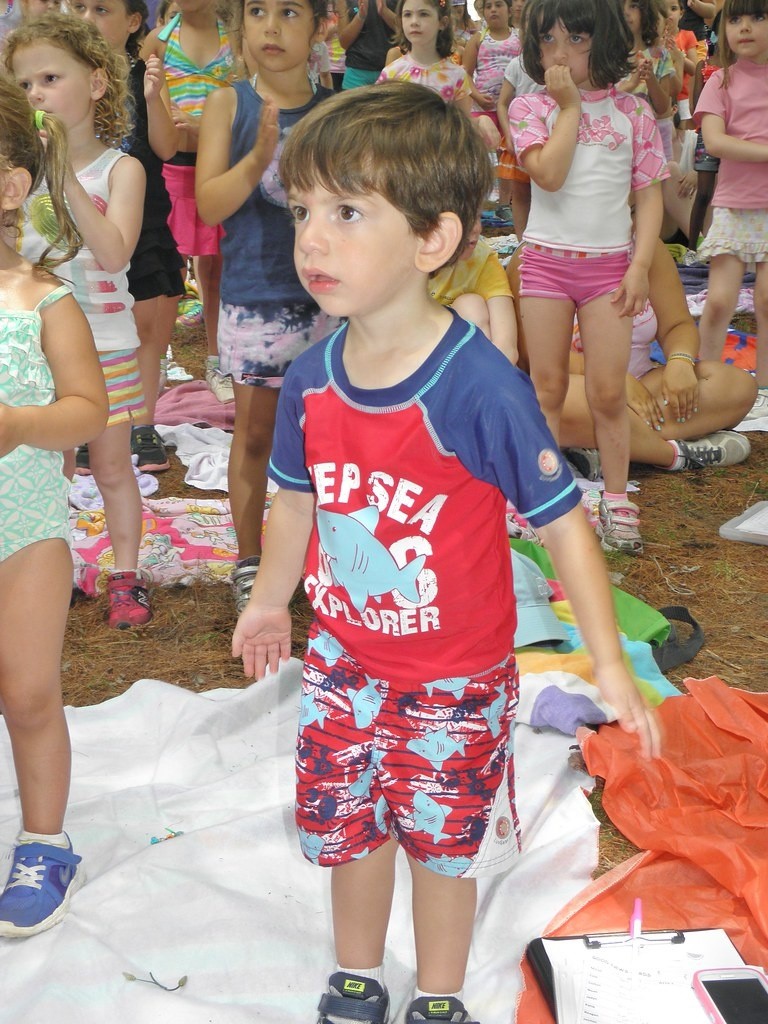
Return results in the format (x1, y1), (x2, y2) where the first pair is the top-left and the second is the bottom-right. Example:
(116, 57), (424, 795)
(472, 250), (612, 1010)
(1, 10), (153, 627)
(0, 0), (768, 474)
(508, 1), (670, 557)
(231, 82), (663, 1024)
(195, 0), (341, 623)
(0, 76), (110, 941)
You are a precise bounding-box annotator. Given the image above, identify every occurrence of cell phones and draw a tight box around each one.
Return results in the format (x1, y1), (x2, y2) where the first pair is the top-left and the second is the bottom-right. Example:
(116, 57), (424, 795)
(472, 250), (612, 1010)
(693, 967), (768, 1024)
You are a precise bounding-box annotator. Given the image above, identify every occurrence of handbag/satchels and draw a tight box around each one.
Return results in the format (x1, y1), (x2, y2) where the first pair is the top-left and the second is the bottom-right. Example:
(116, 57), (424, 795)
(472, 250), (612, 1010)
(610, 585), (704, 673)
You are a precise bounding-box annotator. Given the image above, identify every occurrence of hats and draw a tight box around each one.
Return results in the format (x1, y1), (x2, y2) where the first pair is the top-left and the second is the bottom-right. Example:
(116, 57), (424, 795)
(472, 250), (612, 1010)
(511, 547), (570, 648)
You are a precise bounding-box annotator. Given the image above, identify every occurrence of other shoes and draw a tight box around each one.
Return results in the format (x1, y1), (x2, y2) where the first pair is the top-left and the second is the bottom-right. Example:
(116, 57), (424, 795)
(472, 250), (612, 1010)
(154, 360), (169, 396)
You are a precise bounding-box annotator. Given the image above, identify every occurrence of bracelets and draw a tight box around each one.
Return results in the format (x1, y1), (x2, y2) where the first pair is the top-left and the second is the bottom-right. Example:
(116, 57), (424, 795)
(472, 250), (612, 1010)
(670, 353), (700, 367)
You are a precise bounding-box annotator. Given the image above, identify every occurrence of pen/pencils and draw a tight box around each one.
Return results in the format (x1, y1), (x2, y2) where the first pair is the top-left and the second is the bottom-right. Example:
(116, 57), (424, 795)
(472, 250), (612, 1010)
(630, 898), (642, 981)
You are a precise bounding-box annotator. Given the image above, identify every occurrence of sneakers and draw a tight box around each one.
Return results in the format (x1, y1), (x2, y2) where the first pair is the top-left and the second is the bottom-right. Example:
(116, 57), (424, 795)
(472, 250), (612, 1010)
(673, 431), (751, 471)
(175, 301), (205, 328)
(506, 505), (539, 544)
(75, 444), (92, 475)
(566, 447), (602, 482)
(182, 280), (200, 299)
(230, 556), (266, 617)
(581, 489), (602, 526)
(205, 367), (236, 403)
(129, 426), (170, 470)
(495, 204), (515, 221)
(317, 972), (390, 1024)
(594, 500), (644, 557)
(0, 831), (87, 937)
(730, 389), (768, 432)
(404, 996), (480, 1024)
(105, 571), (153, 629)
